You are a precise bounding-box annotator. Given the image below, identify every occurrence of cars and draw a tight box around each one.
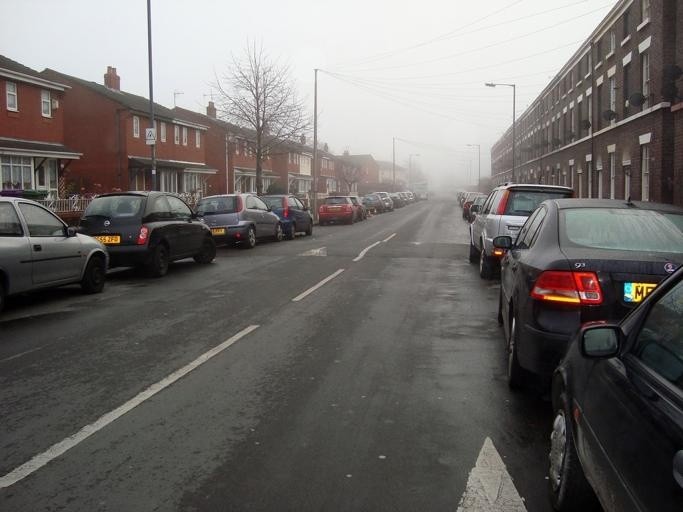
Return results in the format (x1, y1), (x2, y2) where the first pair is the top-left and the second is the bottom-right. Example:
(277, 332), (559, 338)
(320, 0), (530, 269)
(261, 193), (315, 237)
(193, 192), (282, 248)
(319, 190), (417, 225)
(0, 197), (110, 298)
(78, 191), (217, 277)
(493, 199), (682, 392)
(456, 192), (489, 221)
(548, 266), (681, 512)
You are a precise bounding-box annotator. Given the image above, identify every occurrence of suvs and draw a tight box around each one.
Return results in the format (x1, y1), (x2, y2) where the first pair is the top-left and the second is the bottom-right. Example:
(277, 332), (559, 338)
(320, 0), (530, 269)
(469, 183), (576, 280)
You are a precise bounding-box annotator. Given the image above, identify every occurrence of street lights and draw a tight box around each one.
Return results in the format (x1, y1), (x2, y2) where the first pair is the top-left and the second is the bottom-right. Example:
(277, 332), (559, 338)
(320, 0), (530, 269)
(467, 144), (480, 190)
(407, 154), (419, 188)
(486, 84), (516, 181)
